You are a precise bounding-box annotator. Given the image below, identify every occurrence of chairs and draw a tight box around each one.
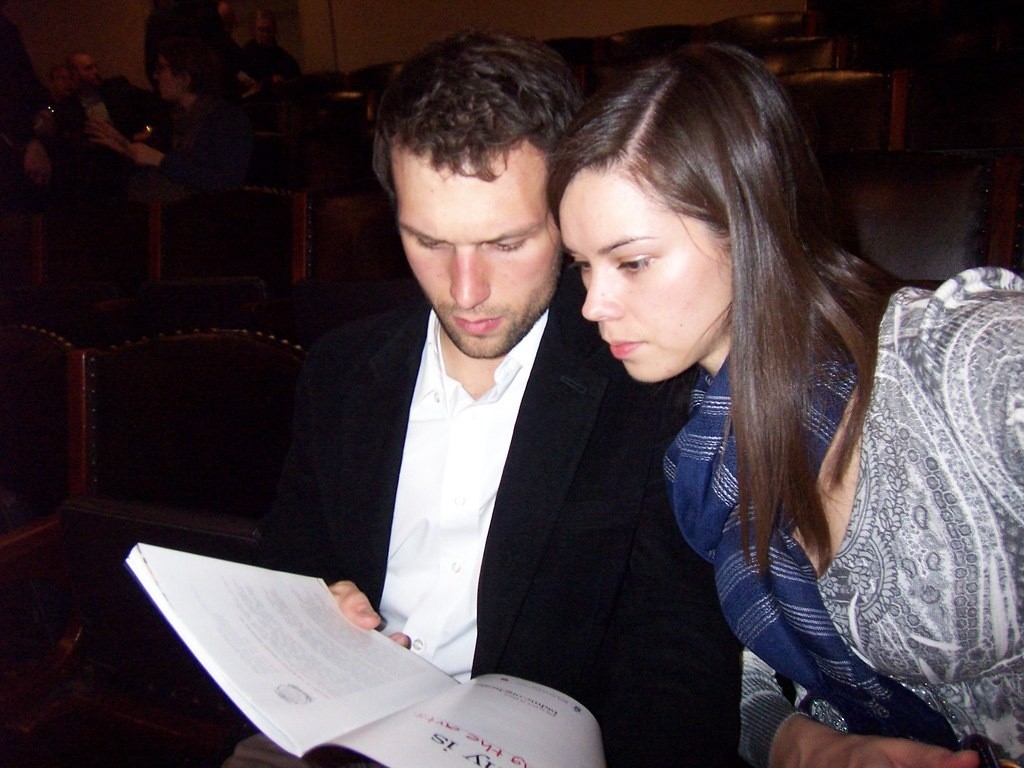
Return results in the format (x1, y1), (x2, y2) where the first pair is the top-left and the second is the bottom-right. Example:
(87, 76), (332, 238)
(0, 0), (432, 768)
(550, 0), (1024, 298)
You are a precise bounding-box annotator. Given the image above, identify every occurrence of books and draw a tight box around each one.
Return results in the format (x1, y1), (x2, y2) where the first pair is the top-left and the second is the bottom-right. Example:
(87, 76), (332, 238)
(123, 543), (606, 768)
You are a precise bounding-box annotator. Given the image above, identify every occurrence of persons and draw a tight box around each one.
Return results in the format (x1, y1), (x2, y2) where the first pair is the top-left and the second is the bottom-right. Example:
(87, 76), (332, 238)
(0, 0), (1024, 768)
